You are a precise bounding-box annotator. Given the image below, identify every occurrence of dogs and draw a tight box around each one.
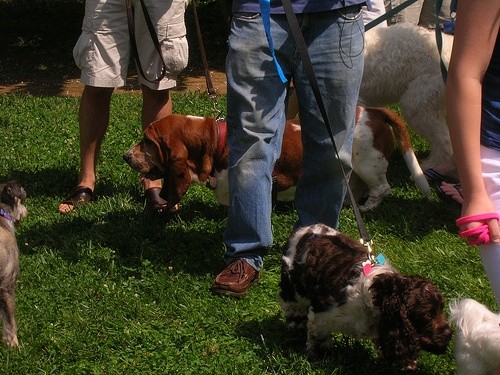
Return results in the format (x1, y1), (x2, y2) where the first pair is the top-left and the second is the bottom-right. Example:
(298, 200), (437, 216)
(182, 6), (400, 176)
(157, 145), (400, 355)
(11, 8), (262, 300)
(0, 182), (30, 347)
(280, 222), (453, 375)
(448, 297), (500, 375)
(284, 22), (458, 180)
(121, 114), (434, 213)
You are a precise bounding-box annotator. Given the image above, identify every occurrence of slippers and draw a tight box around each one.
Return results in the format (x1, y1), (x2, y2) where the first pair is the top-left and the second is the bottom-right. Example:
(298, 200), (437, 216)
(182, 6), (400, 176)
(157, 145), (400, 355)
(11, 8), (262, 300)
(434, 182), (466, 211)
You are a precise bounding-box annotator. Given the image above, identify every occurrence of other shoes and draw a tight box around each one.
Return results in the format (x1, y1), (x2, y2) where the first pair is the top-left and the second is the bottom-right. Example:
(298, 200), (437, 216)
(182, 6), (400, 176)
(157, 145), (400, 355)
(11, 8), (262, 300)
(57, 186), (94, 214)
(144, 187), (168, 207)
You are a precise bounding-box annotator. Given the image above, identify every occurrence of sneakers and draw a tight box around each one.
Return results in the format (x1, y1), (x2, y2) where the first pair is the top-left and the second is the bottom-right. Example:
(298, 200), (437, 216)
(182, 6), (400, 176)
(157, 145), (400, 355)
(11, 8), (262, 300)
(210, 258), (260, 298)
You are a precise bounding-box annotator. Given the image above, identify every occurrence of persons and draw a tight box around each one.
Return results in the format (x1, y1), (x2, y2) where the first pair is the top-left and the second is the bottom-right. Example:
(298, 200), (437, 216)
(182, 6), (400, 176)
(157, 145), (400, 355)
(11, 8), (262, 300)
(211, 0), (373, 299)
(58, 0), (191, 214)
(362, 0), (500, 309)
